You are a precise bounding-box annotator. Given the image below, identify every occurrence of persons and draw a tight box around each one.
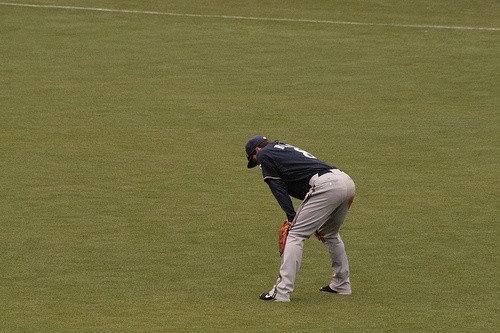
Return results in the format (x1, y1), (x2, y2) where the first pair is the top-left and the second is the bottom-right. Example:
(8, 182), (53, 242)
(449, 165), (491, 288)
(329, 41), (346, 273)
(244, 135), (355, 302)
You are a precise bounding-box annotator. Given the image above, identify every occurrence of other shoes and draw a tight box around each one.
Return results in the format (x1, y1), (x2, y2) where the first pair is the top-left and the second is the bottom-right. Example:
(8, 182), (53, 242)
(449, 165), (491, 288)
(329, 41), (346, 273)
(319, 285), (339, 293)
(259, 293), (272, 300)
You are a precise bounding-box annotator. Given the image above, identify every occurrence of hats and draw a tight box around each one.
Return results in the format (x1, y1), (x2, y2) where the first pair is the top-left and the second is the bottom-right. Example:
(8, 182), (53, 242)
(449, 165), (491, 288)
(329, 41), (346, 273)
(245, 135), (269, 169)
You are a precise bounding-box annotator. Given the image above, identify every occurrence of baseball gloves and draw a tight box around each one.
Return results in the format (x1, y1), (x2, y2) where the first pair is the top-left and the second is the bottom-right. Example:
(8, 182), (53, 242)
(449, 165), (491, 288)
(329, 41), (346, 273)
(278, 220), (292, 256)
